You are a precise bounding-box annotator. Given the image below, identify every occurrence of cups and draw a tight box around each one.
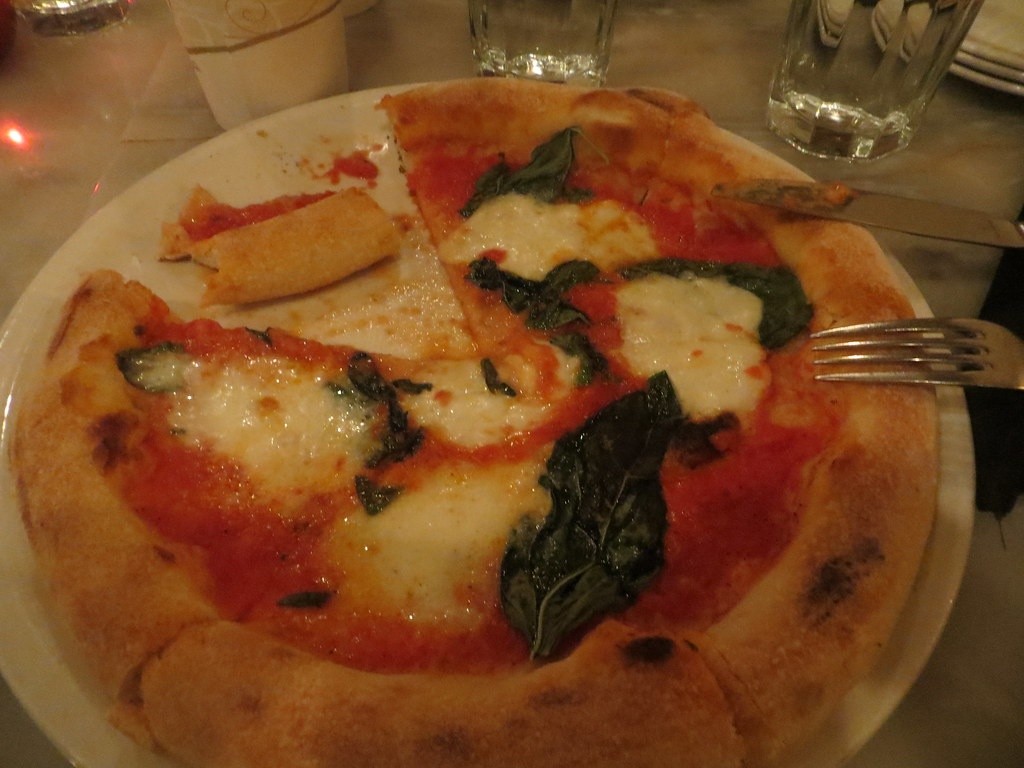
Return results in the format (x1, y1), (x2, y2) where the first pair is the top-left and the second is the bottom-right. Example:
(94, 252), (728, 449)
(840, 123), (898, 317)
(167, 0), (350, 131)
(467, 0), (618, 85)
(12, 0), (128, 34)
(765, 0), (982, 160)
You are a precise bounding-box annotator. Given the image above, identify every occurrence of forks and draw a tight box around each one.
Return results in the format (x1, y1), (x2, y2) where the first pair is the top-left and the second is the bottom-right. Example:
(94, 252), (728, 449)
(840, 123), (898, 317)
(809, 316), (1024, 389)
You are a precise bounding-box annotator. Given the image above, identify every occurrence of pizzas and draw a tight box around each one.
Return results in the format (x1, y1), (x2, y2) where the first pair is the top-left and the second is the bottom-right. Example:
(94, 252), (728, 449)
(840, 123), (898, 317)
(9, 75), (943, 768)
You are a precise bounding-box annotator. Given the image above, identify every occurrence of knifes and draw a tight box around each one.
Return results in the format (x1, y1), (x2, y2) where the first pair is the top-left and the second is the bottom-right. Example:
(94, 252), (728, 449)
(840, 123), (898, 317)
(710, 178), (1024, 248)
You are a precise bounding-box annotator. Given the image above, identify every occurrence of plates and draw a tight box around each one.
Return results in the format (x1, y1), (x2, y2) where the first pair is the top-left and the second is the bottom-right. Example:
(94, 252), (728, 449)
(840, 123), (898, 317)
(817, 0), (1024, 94)
(0, 82), (978, 768)
